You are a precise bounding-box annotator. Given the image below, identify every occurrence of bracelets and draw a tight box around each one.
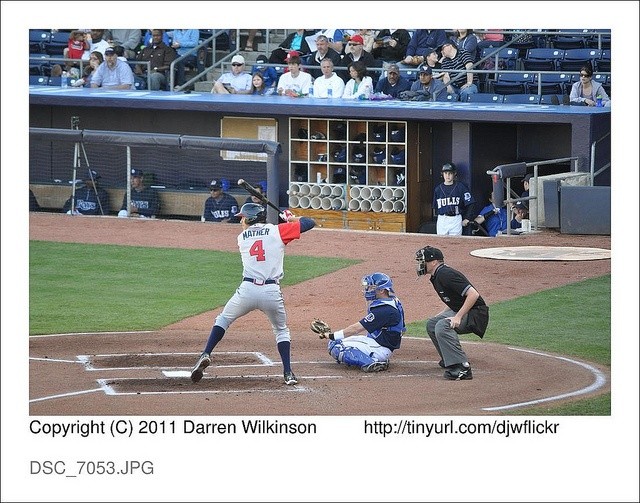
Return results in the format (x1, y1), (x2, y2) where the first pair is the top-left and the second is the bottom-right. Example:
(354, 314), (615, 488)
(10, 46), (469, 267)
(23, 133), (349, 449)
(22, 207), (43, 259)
(334, 328), (345, 340)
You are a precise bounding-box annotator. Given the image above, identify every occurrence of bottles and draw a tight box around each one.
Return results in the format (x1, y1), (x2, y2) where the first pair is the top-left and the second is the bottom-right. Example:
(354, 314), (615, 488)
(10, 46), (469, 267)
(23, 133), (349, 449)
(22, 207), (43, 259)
(328, 83), (332, 98)
(309, 82), (313, 97)
(61, 71), (67, 87)
(597, 95), (603, 107)
(40, 34), (47, 59)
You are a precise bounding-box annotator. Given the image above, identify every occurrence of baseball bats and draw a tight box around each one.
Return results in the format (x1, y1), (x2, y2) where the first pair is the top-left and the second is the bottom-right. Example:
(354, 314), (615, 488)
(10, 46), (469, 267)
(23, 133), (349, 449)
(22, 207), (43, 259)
(237, 179), (284, 214)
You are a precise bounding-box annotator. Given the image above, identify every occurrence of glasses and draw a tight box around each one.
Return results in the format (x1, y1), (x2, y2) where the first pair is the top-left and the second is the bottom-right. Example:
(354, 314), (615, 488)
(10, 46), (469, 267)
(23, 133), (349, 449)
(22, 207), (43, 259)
(211, 189), (219, 192)
(579, 73), (591, 78)
(348, 43), (361, 46)
(441, 45), (450, 49)
(231, 63), (243, 66)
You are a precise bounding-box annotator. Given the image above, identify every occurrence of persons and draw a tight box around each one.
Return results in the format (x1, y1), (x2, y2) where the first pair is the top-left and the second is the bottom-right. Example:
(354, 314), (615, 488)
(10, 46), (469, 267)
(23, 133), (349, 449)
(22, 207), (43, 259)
(143, 28), (169, 46)
(342, 61), (373, 99)
(104, 28), (142, 58)
(90, 46), (136, 89)
(313, 57), (345, 97)
(117, 168), (160, 219)
(276, 55), (311, 95)
(191, 203), (315, 386)
(210, 54), (254, 93)
(510, 200), (527, 228)
(245, 29), (257, 51)
(416, 245), (489, 381)
(171, 28), (200, 91)
(473, 190), (510, 236)
(432, 162), (476, 235)
(134, 28), (179, 90)
(437, 39), (480, 101)
(68, 30), (90, 59)
(81, 29), (110, 59)
(551, 65), (609, 106)
(60, 170), (110, 215)
(244, 183), (267, 207)
(251, 54), (278, 86)
(320, 271), (405, 372)
(251, 72), (276, 94)
(374, 64), (411, 99)
(81, 50), (103, 87)
(201, 178), (241, 223)
(410, 65), (447, 101)
(520, 173), (534, 208)
(267, 29), (479, 70)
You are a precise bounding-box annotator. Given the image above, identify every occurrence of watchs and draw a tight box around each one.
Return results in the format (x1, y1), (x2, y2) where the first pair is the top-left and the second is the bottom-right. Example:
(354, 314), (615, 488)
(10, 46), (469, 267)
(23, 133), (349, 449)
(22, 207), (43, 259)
(154, 66), (158, 71)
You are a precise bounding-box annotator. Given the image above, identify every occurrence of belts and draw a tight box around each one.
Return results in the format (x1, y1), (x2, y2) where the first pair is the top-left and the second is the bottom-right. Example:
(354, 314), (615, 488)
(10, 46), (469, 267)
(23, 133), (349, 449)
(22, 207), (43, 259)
(440, 213), (461, 216)
(244, 277), (278, 285)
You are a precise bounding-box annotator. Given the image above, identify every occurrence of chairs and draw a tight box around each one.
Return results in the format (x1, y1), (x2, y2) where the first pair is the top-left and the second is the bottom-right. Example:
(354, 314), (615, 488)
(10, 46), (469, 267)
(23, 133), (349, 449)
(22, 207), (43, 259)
(564, 74), (607, 94)
(526, 74), (572, 94)
(398, 62), (417, 79)
(48, 76), (72, 87)
(555, 49), (602, 71)
(491, 73), (535, 94)
(29, 29), (51, 55)
(585, 29), (611, 49)
(438, 92), (458, 102)
(549, 29), (592, 48)
(41, 55), (64, 75)
(541, 94), (569, 104)
(467, 93), (503, 103)
(30, 74), (48, 87)
(520, 48), (565, 71)
(43, 32), (70, 53)
(480, 47), (519, 69)
(503, 94), (540, 104)
(592, 49), (611, 72)
(29, 51), (50, 75)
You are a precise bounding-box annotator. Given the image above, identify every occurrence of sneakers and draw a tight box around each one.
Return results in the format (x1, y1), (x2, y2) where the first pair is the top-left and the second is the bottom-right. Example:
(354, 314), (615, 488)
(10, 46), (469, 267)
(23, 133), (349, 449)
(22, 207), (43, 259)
(191, 355), (211, 382)
(284, 372), (297, 384)
(366, 360), (389, 373)
(444, 363), (472, 381)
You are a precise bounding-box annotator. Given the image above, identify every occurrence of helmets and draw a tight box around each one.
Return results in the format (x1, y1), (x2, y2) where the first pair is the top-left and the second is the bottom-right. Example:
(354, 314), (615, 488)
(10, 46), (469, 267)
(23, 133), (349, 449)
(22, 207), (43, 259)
(361, 272), (395, 300)
(415, 246), (444, 278)
(442, 163), (457, 172)
(130, 169), (143, 176)
(234, 204), (268, 224)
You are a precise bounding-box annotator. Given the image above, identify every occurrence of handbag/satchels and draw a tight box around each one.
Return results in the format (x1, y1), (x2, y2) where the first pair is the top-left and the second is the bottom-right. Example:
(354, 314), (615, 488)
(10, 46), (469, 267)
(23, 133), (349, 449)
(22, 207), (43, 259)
(466, 305), (489, 340)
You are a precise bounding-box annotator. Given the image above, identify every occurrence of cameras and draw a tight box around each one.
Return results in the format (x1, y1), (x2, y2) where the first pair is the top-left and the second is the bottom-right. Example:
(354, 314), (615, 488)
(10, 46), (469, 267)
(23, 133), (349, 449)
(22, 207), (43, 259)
(72, 116), (80, 126)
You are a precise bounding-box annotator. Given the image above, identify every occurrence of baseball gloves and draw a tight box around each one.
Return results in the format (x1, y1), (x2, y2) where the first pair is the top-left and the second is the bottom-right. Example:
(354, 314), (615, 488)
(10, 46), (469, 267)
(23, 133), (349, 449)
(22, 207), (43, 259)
(309, 317), (333, 339)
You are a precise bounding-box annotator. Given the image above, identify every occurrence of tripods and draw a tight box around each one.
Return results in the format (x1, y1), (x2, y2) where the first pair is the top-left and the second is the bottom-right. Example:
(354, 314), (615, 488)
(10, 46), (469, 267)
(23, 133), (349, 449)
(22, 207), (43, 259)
(71, 126), (104, 215)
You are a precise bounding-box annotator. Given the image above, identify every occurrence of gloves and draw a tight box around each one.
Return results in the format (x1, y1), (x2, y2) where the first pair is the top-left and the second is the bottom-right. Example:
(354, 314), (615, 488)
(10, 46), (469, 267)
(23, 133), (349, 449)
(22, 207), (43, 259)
(279, 210), (294, 221)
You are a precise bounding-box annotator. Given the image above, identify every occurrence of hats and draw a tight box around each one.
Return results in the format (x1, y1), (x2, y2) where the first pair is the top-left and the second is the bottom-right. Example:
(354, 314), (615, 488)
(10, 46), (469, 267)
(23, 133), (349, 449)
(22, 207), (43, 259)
(521, 175), (534, 182)
(423, 47), (437, 56)
(208, 180), (221, 189)
(104, 47), (115, 54)
(287, 56), (300, 64)
(84, 170), (101, 179)
(347, 35), (363, 45)
(387, 64), (400, 74)
(283, 51), (300, 61)
(439, 39), (454, 47)
(419, 66), (432, 75)
(512, 201), (527, 209)
(255, 55), (268, 69)
(231, 55), (245, 64)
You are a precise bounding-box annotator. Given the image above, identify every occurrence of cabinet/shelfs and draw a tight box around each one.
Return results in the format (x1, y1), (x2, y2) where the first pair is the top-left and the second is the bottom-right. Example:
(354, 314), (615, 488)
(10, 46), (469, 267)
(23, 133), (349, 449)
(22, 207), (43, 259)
(288, 116), (408, 187)
(290, 207), (344, 229)
(346, 211), (406, 232)
